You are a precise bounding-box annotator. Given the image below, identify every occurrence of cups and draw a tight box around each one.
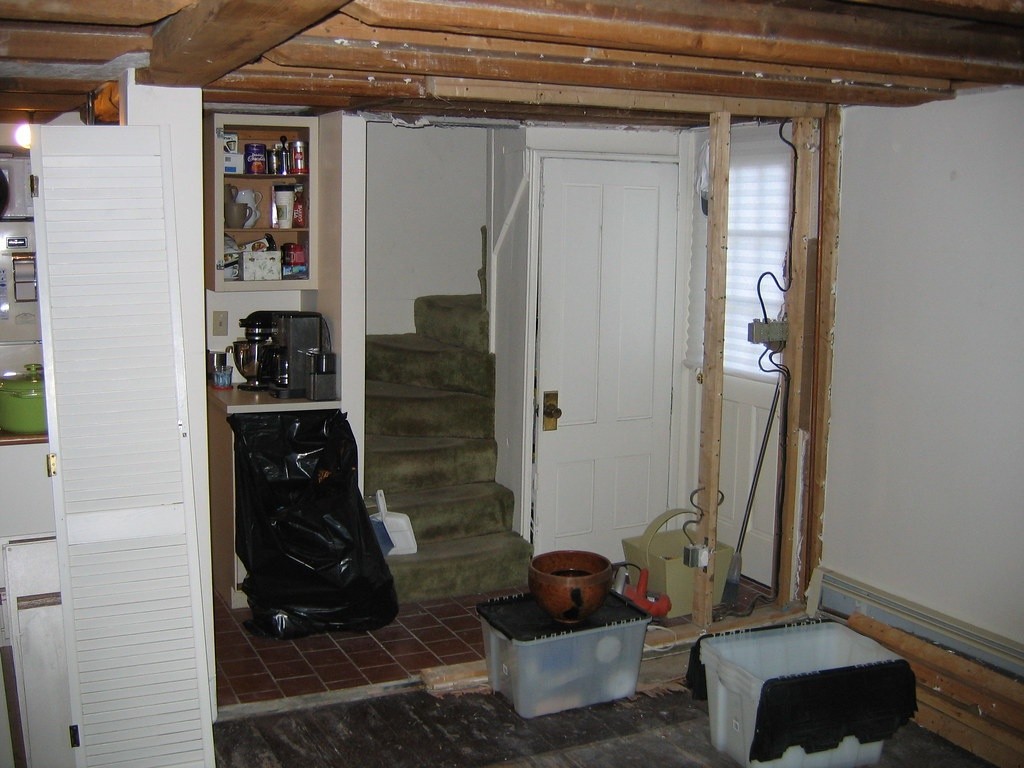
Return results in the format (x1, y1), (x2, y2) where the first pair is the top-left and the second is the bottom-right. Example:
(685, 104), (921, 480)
(224, 203), (252, 229)
(213, 366), (232, 388)
(274, 185), (295, 229)
(224, 184), (238, 203)
(235, 189), (263, 209)
(267, 149), (278, 174)
(244, 209), (260, 228)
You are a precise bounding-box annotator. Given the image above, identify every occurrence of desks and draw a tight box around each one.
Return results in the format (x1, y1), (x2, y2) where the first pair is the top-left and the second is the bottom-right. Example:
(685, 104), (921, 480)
(208, 385), (340, 611)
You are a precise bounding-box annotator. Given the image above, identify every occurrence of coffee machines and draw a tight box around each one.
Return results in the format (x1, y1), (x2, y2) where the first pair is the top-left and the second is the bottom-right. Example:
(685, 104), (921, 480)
(233, 310), (327, 398)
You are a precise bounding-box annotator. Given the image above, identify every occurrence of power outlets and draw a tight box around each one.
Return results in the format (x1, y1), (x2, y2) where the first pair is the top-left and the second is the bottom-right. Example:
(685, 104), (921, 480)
(212, 310), (229, 336)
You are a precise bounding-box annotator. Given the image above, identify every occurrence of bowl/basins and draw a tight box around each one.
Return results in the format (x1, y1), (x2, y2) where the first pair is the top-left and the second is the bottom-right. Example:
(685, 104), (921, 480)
(529, 551), (611, 623)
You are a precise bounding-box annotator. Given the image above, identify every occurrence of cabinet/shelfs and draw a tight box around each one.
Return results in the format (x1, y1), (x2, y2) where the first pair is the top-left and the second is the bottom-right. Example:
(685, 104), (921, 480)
(203, 112), (318, 293)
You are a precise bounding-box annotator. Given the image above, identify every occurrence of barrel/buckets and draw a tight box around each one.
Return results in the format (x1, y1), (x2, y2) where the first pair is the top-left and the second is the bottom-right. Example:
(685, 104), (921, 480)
(288, 141), (307, 173)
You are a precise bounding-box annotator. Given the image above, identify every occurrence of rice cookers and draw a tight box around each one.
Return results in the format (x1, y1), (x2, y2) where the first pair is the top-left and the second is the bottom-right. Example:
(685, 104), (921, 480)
(0, 362), (48, 435)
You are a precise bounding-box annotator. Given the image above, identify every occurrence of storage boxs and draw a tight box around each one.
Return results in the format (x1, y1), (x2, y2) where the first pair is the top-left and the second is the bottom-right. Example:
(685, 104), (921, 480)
(688, 612), (918, 768)
(240, 250), (283, 280)
(476, 590), (653, 718)
(223, 154), (243, 174)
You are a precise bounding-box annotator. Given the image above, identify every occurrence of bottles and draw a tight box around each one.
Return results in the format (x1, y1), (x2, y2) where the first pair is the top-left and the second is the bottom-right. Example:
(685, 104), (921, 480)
(293, 192), (305, 228)
(279, 136), (291, 173)
